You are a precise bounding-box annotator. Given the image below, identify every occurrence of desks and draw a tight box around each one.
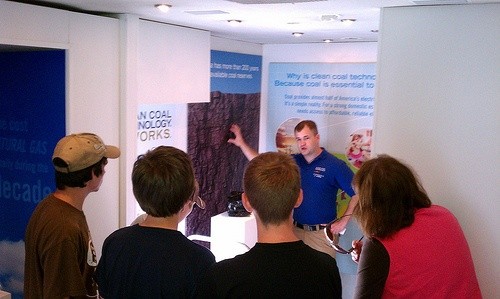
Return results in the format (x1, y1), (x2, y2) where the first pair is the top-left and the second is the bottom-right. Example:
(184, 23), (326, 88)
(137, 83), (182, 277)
(210, 212), (259, 263)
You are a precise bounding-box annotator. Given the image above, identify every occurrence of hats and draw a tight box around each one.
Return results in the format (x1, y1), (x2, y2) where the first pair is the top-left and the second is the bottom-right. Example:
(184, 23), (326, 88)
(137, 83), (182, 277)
(188, 179), (207, 210)
(52, 133), (121, 173)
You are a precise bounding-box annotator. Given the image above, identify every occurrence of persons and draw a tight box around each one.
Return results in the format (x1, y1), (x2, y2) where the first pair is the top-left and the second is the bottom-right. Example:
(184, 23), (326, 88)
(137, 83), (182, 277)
(198, 152), (342, 299)
(226, 119), (361, 259)
(23, 132), (109, 299)
(94, 145), (217, 299)
(130, 176), (199, 227)
(351, 154), (483, 299)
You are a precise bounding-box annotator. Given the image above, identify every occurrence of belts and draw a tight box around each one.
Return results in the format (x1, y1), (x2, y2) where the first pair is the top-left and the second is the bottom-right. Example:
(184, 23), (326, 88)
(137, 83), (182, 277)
(294, 221), (326, 231)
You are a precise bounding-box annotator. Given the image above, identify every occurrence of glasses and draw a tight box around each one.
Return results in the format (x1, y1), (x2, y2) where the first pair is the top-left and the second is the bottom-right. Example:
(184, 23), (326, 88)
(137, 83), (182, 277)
(324, 214), (365, 254)
